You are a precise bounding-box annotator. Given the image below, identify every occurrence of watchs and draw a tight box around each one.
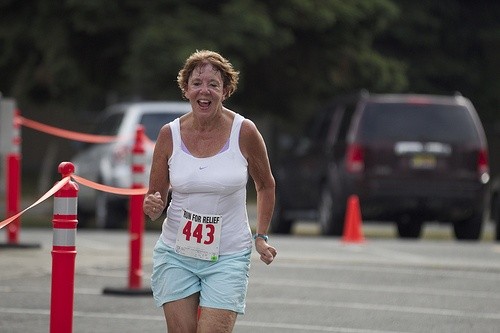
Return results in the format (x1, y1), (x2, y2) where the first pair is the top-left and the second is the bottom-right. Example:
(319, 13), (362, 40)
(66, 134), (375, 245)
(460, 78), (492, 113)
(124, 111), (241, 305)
(254, 233), (268, 243)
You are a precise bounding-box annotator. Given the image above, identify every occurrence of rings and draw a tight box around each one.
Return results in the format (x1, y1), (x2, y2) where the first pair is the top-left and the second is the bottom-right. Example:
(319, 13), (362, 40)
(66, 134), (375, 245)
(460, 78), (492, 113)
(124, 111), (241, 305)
(152, 207), (155, 213)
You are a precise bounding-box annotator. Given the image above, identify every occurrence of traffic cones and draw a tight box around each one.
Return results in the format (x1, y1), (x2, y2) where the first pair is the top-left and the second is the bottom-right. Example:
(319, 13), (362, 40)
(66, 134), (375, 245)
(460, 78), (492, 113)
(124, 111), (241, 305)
(342, 195), (365, 244)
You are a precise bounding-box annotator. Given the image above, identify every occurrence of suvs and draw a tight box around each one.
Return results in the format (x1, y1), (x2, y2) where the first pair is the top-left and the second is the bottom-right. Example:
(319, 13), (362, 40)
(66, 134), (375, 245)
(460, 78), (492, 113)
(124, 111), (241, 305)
(69, 99), (195, 231)
(260, 89), (490, 243)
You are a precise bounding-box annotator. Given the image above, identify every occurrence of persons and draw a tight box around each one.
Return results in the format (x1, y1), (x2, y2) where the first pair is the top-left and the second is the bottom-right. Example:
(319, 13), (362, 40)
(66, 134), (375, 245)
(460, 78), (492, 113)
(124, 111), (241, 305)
(143, 49), (278, 333)
